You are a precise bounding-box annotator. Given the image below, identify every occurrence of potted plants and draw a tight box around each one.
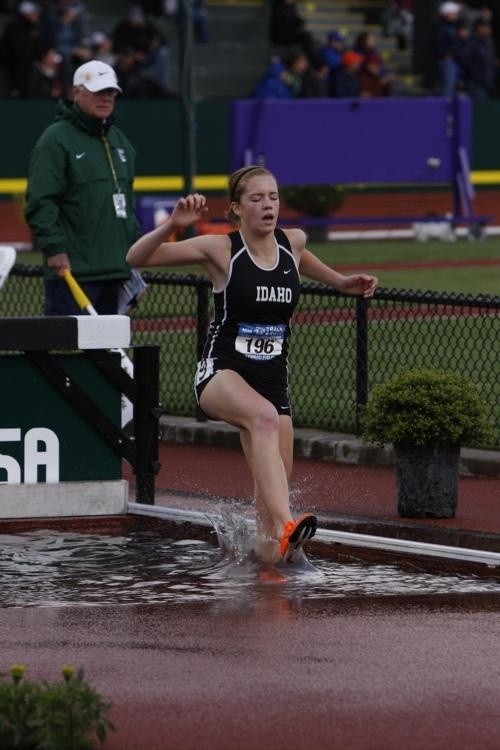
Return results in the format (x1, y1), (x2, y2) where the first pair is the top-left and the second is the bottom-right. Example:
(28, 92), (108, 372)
(355, 358), (497, 523)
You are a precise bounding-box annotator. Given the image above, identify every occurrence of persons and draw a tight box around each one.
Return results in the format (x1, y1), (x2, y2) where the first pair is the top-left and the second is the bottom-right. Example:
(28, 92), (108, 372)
(22, 59), (144, 317)
(379, 1), (499, 105)
(252, 0), (394, 104)
(124, 165), (378, 565)
(1, 1), (211, 104)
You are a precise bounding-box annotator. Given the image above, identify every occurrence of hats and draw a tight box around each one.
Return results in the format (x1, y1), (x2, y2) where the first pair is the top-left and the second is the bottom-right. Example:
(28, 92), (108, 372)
(73, 60), (123, 94)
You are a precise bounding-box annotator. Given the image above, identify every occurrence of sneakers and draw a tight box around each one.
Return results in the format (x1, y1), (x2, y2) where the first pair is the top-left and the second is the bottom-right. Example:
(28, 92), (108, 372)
(272, 512), (317, 564)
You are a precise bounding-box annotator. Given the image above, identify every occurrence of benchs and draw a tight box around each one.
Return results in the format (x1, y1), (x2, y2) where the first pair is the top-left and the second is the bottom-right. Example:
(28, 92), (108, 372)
(2, 1), (324, 107)
